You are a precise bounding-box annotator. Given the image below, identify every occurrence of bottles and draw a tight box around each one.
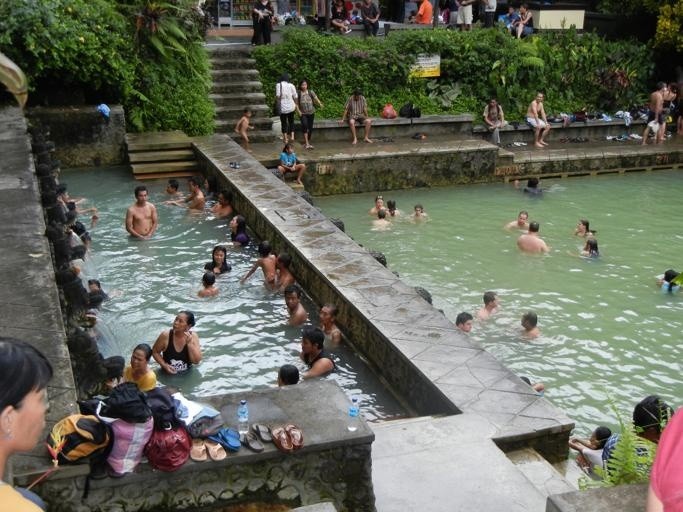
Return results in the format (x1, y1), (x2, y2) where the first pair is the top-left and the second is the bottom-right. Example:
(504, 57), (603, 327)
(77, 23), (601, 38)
(347, 397), (359, 430)
(238, 399), (248, 432)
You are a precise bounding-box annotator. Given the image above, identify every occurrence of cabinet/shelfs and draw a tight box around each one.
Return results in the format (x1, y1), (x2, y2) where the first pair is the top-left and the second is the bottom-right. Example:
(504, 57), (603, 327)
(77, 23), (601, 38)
(217, 0), (318, 28)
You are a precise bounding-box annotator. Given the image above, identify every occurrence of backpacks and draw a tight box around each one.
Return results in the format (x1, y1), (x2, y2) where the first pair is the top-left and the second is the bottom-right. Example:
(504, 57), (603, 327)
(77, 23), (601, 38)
(47, 411), (113, 466)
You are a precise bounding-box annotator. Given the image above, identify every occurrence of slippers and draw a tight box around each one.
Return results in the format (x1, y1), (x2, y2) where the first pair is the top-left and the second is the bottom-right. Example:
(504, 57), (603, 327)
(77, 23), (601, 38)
(249, 421), (272, 441)
(238, 430), (265, 452)
(207, 439), (227, 462)
(268, 423), (294, 453)
(209, 427), (242, 452)
(188, 439), (207, 461)
(285, 423), (305, 449)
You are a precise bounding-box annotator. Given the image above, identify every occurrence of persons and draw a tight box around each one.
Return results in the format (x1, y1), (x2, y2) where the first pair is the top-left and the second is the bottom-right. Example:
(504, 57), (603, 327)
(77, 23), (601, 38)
(480, 96), (509, 148)
(455, 291), (540, 339)
(0, 336), (52, 510)
(514, 177), (541, 193)
(293, 79), (324, 149)
(520, 376), (545, 390)
(565, 424), (608, 478)
(272, 71), (301, 145)
(336, 89), (373, 145)
(234, 105), (257, 155)
(576, 219), (599, 256)
(508, 211), (549, 253)
(369, 194), (427, 225)
(661, 269), (681, 293)
(642, 80), (683, 146)
(630, 394), (676, 447)
(246, 0), (537, 46)
(50, 166), (344, 391)
(277, 143), (305, 186)
(526, 90), (551, 149)
(557, 107), (589, 128)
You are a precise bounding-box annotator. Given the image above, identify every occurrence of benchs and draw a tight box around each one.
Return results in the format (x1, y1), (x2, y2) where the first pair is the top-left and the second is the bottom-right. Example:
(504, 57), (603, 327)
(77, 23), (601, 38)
(338, 23), (379, 40)
(383, 22), (433, 37)
